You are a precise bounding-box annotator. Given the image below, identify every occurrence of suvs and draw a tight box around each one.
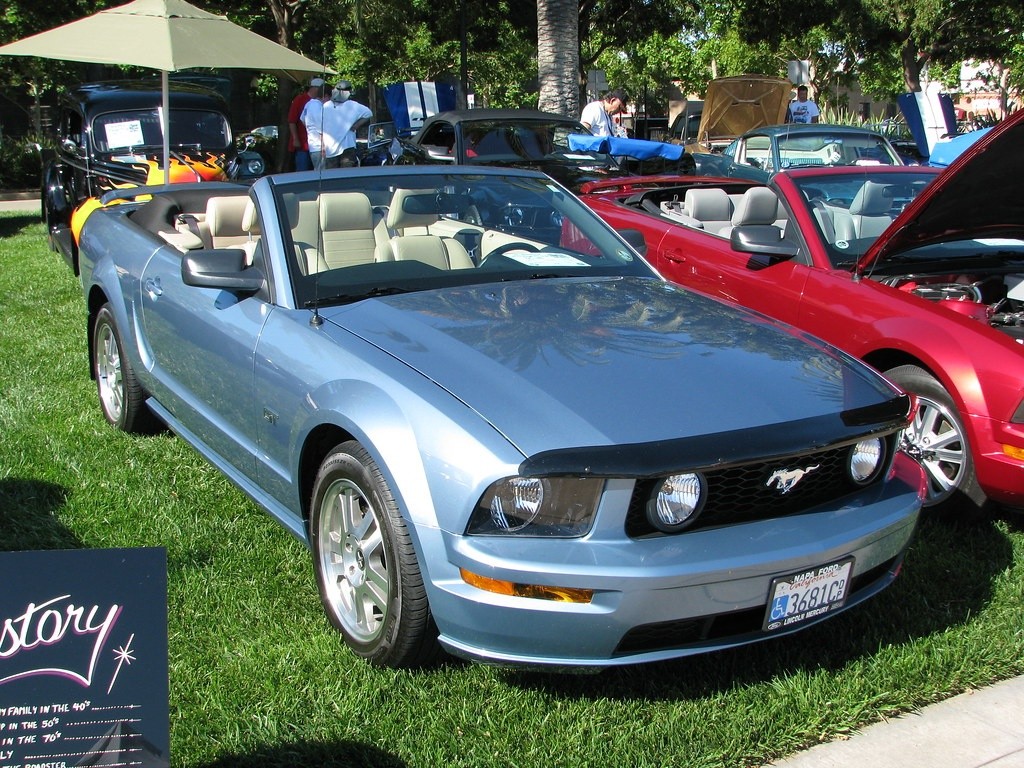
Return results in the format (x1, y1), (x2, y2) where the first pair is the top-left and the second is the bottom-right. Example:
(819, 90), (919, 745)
(41, 80), (265, 278)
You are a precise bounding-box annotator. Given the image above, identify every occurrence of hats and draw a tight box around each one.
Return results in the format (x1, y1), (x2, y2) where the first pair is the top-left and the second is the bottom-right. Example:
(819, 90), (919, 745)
(612, 89), (630, 114)
(311, 78), (328, 87)
(332, 81), (351, 103)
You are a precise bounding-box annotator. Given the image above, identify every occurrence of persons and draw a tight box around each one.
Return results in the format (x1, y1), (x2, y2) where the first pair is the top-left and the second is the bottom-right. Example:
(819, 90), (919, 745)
(288, 78), (373, 171)
(789, 86), (820, 124)
(450, 133), (480, 159)
(579, 89), (629, 137)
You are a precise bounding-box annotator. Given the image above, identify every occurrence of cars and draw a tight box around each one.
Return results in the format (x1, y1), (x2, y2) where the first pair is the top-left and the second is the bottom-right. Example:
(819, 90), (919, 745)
(352, 82), (661, 247)
(560, 110), (1024, 519)
(623, 74), (994, 220)
(78, 164), (928, 667)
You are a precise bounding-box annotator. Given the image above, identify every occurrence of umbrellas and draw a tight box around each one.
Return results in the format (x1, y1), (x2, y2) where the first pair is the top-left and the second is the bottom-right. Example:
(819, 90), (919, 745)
(0, 0), (336, 185)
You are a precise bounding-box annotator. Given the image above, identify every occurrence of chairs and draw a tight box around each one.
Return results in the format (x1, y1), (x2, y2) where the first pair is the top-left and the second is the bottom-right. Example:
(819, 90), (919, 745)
(377, 189), (476, 270)
(317, 192), (389, 271)
(227, 194), (330, 287)
(720, 187), (778, 239)
(844, 181), (893, 242)
(685, 188), (730, 234)
(180, 196), (260, 249)
